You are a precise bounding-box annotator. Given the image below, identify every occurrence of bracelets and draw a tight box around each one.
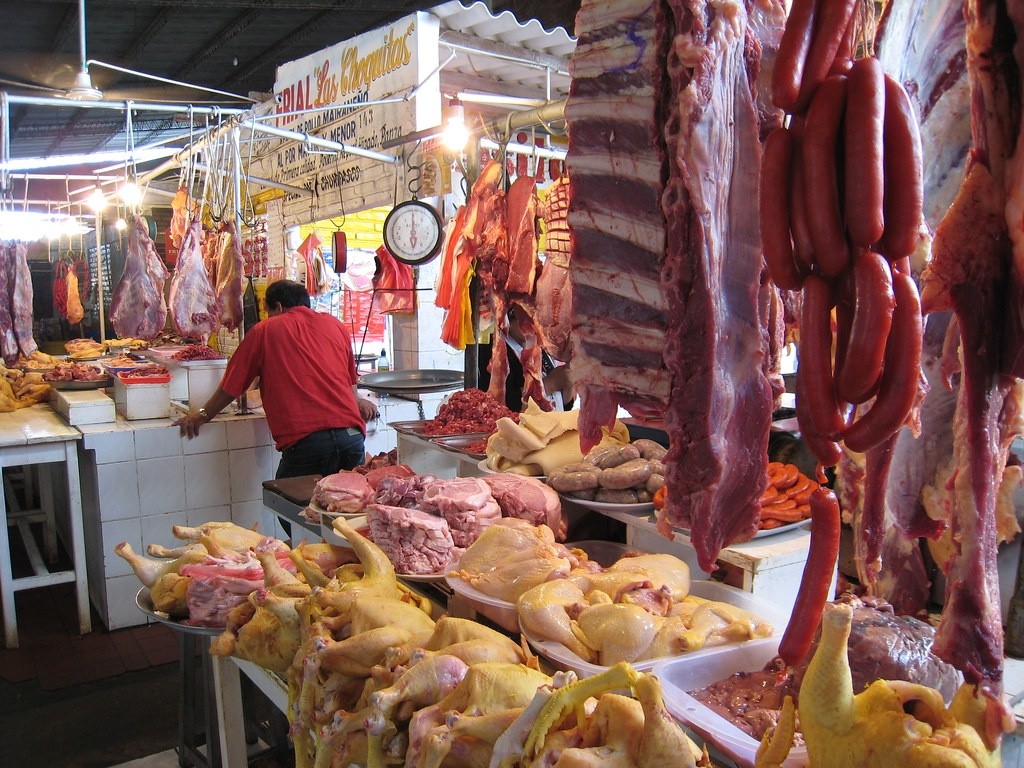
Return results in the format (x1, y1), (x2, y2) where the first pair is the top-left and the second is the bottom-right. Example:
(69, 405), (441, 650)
(198, 407), (211, 421)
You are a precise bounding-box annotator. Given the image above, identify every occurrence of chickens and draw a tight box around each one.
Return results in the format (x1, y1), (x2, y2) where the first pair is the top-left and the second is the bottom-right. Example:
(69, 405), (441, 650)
(115, 517), (1017, 768)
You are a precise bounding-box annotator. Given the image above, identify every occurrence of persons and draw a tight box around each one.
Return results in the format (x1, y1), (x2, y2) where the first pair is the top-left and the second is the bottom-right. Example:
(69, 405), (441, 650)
(171, 280), (367, 539)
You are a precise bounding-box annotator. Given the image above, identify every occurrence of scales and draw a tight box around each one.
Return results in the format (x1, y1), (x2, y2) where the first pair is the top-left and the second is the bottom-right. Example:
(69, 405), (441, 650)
(354, 129), (464, 398)
(331, 139), (378, 366)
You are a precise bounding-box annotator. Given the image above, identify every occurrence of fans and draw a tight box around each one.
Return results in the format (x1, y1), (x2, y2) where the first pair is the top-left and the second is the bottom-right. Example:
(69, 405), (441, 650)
(0, 0), (214, 101)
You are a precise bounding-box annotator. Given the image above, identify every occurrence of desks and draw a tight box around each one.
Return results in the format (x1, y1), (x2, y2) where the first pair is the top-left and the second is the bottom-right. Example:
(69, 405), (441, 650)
(0, 404), (96, 648)
(205, 637), (331, 768)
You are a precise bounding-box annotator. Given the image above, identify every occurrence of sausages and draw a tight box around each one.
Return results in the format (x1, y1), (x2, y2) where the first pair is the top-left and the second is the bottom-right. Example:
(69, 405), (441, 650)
(759, 2), (924, 665)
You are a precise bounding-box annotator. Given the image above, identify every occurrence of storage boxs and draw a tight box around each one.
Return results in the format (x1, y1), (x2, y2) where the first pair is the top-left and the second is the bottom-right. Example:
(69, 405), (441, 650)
(444, 541), (810, 768)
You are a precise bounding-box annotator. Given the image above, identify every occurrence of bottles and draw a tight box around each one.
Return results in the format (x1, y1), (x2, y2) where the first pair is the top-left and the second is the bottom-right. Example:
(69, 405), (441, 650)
(377, 350), (390, 373)
